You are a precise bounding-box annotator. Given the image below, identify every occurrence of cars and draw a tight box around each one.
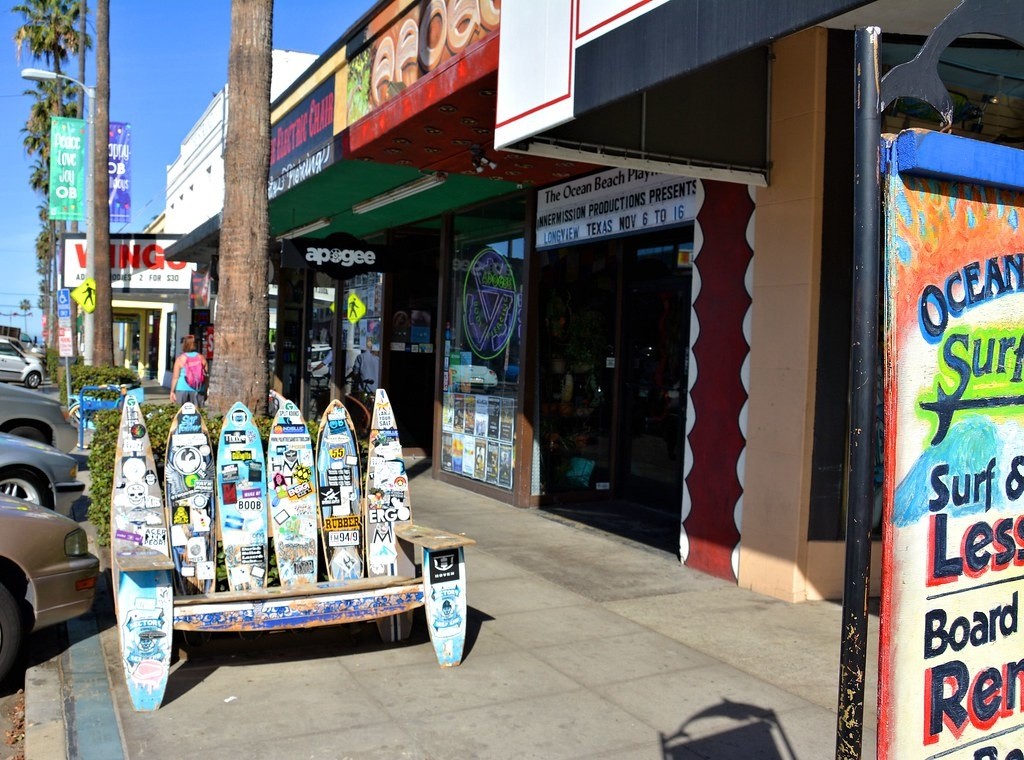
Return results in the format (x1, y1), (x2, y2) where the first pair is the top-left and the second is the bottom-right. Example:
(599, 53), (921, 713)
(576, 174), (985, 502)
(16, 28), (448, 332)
(308, 342), (332, 377)
(1, 494), (100, 686)
(0, 430), (86, 519)
(0, 335), (47, 390)
(0, 381), (79, 453)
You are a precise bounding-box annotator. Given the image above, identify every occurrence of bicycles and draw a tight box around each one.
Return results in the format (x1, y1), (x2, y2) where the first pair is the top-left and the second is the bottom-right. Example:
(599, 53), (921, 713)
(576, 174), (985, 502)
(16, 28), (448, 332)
(309, 365), (376, 423)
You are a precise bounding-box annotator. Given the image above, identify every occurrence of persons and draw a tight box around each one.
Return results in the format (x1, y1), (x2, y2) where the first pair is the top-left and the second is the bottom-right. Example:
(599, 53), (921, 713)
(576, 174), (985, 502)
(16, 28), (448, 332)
(171, 335), (209, 408)
(312, 339), (378, 392)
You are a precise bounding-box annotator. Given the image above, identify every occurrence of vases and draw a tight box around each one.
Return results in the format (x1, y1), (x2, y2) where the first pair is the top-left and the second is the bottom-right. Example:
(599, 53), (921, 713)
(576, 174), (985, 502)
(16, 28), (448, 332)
(544, 316), (565, 336)
(558, 402), (574, 417)
(543, 403), (557, 417)
(552, 358), (567, 374)
(577, 434), (590, 447)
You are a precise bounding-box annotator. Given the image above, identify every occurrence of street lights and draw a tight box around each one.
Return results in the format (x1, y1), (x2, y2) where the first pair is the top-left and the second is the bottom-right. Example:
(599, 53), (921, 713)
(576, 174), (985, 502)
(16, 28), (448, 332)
(22, 66), (96, 368)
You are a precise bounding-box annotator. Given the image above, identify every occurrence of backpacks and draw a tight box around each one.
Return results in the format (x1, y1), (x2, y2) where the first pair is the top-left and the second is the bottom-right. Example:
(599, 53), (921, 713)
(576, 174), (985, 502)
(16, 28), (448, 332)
(181, 353), (205, 389)
